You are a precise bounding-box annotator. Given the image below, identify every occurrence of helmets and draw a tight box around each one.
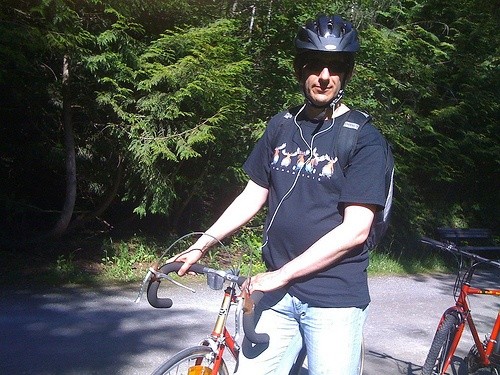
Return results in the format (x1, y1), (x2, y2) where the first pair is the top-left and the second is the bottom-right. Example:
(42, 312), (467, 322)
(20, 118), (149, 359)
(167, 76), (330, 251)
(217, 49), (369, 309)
(293, 12), (361, 54)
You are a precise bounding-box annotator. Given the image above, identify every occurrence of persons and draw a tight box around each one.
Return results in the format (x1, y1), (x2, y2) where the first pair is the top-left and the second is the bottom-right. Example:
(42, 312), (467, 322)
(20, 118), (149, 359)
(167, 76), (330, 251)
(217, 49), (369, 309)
(167, 13), (396, 375)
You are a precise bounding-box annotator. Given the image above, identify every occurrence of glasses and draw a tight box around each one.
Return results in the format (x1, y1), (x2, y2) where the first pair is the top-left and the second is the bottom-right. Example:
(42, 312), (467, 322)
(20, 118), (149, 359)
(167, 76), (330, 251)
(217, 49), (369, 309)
(298, 57), (348, 74)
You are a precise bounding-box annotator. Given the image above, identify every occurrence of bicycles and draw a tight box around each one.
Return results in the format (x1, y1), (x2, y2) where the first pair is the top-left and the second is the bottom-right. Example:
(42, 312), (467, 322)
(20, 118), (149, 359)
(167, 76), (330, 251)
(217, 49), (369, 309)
(134, 231), (309, 375)
(420, 237), (500, 375)
